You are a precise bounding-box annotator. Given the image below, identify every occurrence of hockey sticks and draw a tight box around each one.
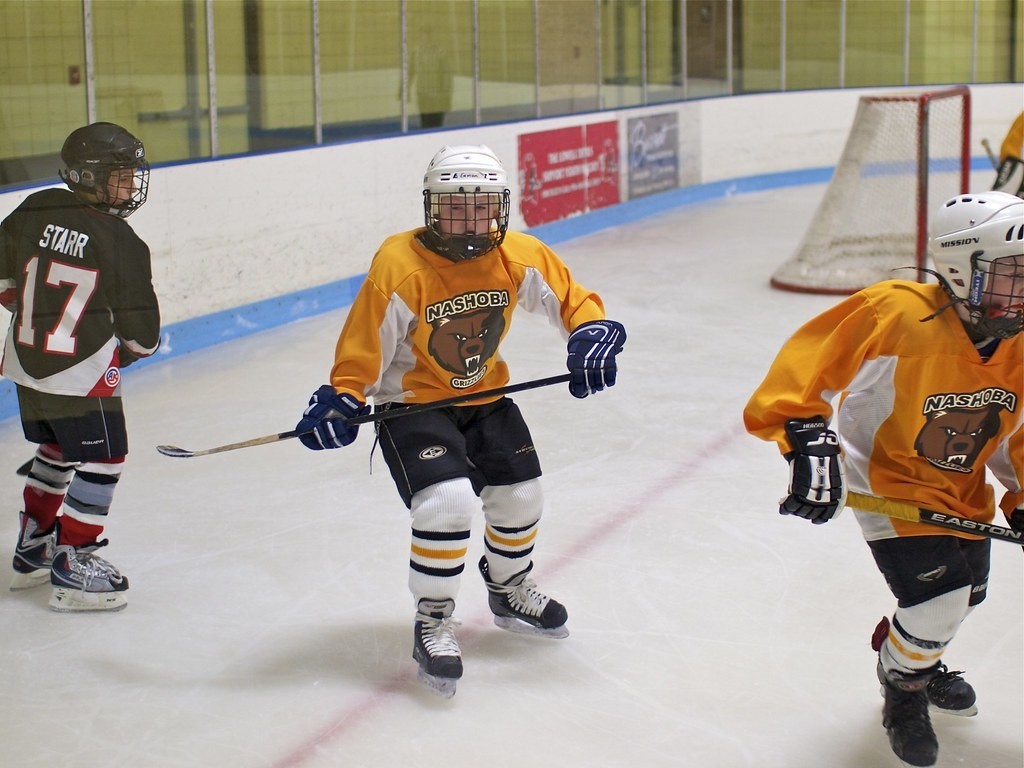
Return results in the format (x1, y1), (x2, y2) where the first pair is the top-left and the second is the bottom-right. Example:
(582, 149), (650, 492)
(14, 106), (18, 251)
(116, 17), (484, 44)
(16, 457), (34, 476)
(980, 137), (998, 171)
(787, 482), (1024, 546)
(156, 360), (618, 459)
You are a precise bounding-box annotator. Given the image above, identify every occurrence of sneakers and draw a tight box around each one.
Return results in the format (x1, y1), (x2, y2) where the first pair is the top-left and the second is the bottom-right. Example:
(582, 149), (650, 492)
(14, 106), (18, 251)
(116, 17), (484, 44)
(413, 597), (464, 700)
(870, 615), (938, 768)
(924, 660), (979, 717)
(49, 517), (130, 612)
(9, 511), (56, 589)
(479, 554), (570, 640)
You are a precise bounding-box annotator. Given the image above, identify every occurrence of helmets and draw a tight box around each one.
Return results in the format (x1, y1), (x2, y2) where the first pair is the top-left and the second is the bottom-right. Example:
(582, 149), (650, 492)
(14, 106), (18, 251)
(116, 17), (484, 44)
(929, 191), (1024, 338)
(58, 122), (150, 219)
(424, 144), (510, 259)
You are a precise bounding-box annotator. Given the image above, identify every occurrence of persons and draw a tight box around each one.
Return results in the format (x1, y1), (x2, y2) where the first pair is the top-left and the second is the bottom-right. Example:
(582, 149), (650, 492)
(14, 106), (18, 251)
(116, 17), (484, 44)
(0, 118), (164, 615)
(396, 23), (453, 129)
(741, 109), (1024, 768)
(294, 140), (627, 700)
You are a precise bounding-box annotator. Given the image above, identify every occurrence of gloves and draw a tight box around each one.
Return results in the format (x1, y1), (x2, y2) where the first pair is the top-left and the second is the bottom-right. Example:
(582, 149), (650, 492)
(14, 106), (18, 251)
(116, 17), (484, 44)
(778, 415), (848, 525)
(567, 320), (627, 398)
(119, 345), (139, 367)
(296, 385), (371, 451)
